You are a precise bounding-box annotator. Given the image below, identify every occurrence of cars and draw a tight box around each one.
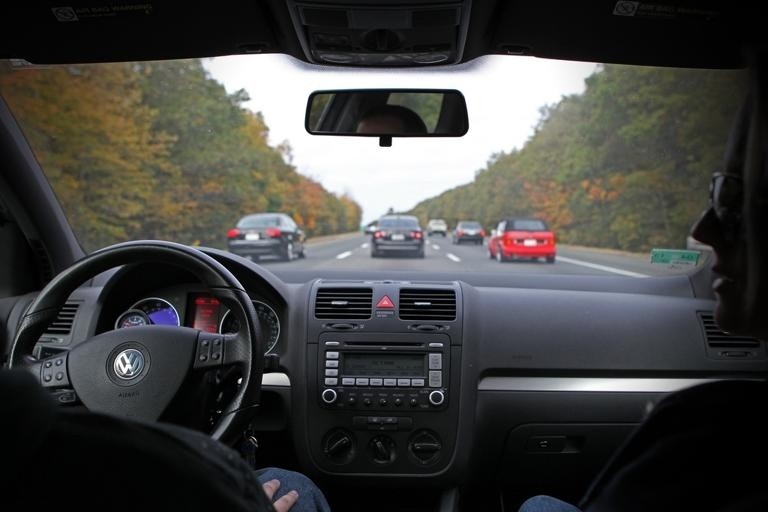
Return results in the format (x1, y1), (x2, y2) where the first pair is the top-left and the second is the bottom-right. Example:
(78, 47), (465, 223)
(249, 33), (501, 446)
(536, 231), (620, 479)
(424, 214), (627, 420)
(371, 214), (423, 259)
(225, 212), (306, 262)
(365, 221), (376, 235)
(425, 219), (448, 236)
(487, 217), (558, 264)
(452, 219), (485, 246)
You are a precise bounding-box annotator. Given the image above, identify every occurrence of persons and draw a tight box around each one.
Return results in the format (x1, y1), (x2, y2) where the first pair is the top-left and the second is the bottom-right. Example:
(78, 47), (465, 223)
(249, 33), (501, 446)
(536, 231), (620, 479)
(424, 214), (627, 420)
(517, 72), (766, 511)
(355, 104), (429, 134)
(0, 312), (332, 512)
